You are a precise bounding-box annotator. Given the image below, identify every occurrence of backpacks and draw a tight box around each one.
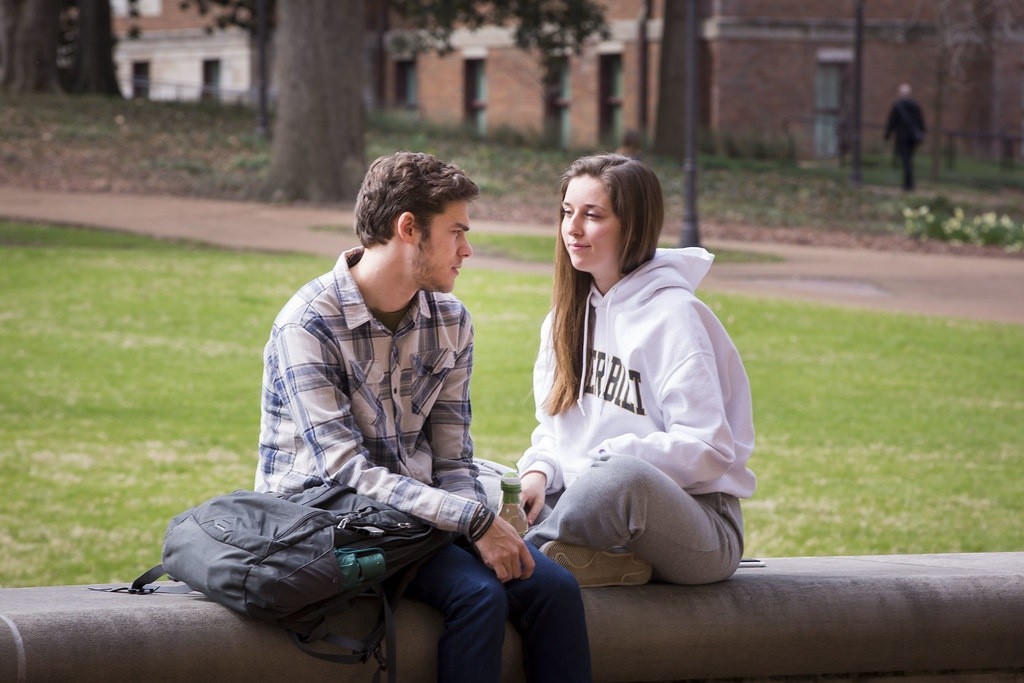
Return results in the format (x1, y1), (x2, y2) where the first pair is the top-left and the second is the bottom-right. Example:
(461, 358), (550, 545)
(162, 482), (465, 628)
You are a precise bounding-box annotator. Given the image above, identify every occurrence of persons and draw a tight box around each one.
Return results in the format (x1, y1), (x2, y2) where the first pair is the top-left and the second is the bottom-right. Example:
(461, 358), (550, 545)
(252, 152), (595, 683)
(475, 154), (756, 590)
(882, 83), (928, 192)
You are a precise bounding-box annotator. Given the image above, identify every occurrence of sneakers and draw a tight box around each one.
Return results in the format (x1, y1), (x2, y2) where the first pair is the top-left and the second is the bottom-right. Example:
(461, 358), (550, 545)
(538, 539), (654, 587)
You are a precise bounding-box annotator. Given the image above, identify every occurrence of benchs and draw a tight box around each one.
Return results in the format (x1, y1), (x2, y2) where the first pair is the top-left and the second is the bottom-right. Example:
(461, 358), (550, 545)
(0, 548), (1024, 682)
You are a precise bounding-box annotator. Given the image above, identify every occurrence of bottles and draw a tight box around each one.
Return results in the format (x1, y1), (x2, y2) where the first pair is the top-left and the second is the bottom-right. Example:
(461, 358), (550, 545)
(496, 471), (529, 538)
